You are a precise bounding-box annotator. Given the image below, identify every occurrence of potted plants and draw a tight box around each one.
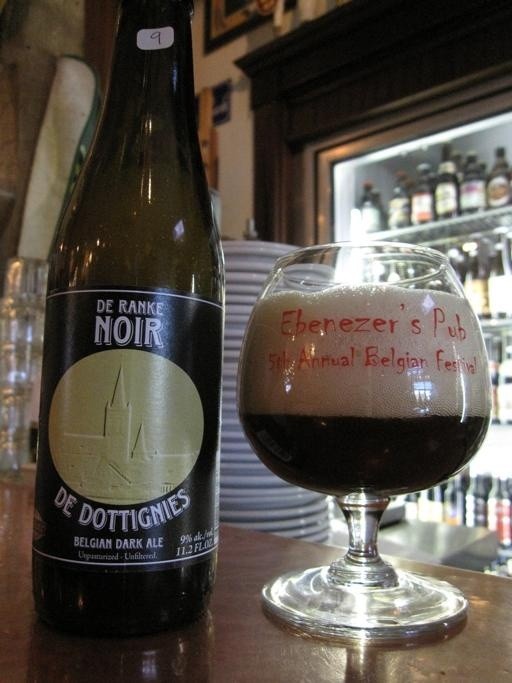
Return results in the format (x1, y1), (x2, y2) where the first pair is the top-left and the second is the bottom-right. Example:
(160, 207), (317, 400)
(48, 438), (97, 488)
(203, 0), (298, 55)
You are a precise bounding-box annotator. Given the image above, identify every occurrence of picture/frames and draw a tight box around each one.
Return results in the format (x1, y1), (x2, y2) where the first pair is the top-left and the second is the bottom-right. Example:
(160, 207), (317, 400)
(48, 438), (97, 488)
(0, 468), (512, 683)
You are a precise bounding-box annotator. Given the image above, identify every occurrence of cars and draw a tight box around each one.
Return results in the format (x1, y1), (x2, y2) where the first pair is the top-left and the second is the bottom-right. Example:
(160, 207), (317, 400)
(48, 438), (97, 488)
(1, 254), (50, 477)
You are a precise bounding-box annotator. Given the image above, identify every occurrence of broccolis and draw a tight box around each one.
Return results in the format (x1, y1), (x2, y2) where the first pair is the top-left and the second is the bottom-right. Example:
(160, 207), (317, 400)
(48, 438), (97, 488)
(295, 64), (511, 584)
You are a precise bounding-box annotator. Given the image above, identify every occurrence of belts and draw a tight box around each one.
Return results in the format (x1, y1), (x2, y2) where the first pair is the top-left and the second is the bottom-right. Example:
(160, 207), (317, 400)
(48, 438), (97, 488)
(249, 240), (493, 636)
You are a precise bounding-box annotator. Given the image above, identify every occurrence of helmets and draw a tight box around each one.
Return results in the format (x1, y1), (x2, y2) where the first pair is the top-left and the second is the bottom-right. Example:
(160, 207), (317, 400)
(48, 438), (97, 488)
(206, 230), (332, 547)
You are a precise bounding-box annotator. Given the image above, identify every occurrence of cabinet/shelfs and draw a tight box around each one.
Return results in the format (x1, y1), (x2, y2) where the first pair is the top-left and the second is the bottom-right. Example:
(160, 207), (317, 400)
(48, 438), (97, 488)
(444, 224), (511, 318)
(387, 456), (510, 555)
(32, 0), (227, 637)
(480, 331), (511, 427)
(348, 139), (511, 231)
